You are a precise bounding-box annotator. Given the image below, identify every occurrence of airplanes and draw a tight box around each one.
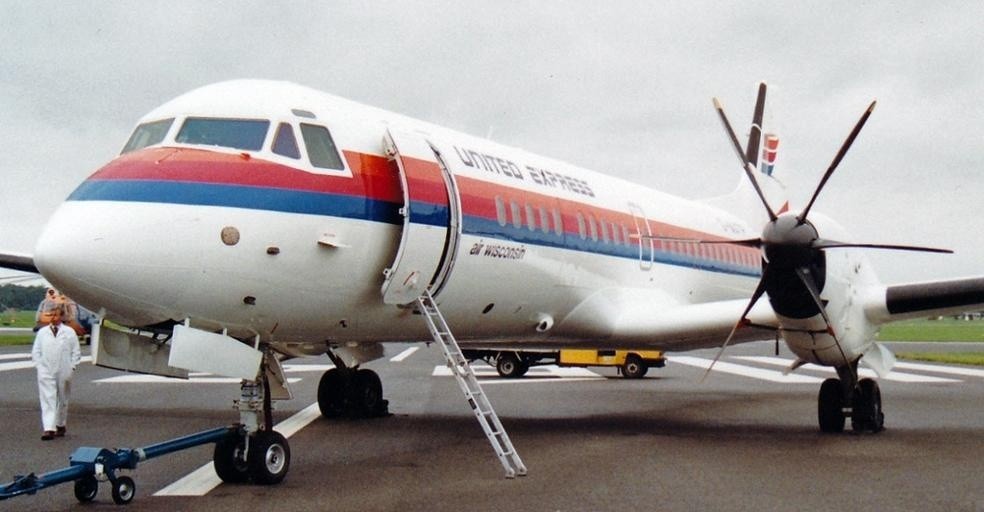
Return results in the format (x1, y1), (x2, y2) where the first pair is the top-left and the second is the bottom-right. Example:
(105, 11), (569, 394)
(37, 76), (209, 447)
(0, 81), (984, 502)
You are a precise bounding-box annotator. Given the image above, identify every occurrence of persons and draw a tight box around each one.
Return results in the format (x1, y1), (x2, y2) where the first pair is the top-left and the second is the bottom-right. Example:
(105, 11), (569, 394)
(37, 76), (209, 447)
(32, 306), (82, 441)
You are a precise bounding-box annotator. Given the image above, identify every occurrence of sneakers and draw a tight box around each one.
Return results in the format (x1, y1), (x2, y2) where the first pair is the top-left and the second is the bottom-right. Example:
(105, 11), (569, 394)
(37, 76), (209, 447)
(41, 430), (53, 439)
(55, 425), (66, 436)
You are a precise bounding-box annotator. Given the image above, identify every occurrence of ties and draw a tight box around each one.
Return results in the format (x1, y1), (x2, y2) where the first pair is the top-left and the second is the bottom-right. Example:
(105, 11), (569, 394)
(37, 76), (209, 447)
(54, 327), (58, 335)
